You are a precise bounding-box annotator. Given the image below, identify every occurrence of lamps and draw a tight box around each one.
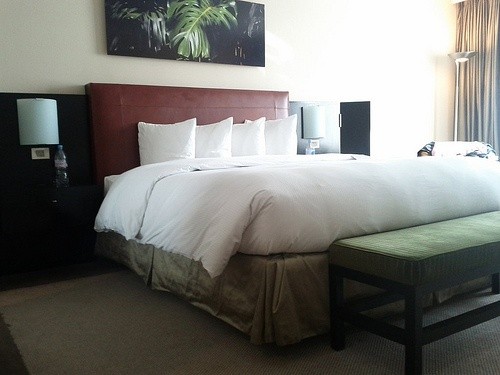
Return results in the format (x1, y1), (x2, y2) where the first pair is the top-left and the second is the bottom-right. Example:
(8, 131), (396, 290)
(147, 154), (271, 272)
(448, 51), (478, 142)
(303, 108), (326, 139)
(17, 99), (59, 145)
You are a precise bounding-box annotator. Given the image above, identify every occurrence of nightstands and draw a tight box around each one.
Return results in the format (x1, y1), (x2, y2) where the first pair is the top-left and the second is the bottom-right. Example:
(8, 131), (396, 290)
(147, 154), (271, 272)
(0, 185), (104, 290)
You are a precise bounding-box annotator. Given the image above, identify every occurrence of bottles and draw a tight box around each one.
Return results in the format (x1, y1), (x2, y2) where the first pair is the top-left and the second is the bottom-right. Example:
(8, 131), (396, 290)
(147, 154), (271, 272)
(305, 139), (315, 155)
(55, 144), (68, 185)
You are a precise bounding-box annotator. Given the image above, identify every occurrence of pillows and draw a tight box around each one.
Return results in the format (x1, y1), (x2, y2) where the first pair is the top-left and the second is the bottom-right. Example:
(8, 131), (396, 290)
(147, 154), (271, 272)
(138, 114), (298, 165)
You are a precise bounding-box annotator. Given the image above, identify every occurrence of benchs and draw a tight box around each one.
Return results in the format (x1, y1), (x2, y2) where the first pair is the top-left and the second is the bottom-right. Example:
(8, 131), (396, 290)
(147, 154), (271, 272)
(329, 210), (500, 375)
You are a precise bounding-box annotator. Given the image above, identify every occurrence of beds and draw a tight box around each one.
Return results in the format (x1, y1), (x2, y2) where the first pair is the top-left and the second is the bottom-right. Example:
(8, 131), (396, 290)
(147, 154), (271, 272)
(85, 83), (500, 358)
